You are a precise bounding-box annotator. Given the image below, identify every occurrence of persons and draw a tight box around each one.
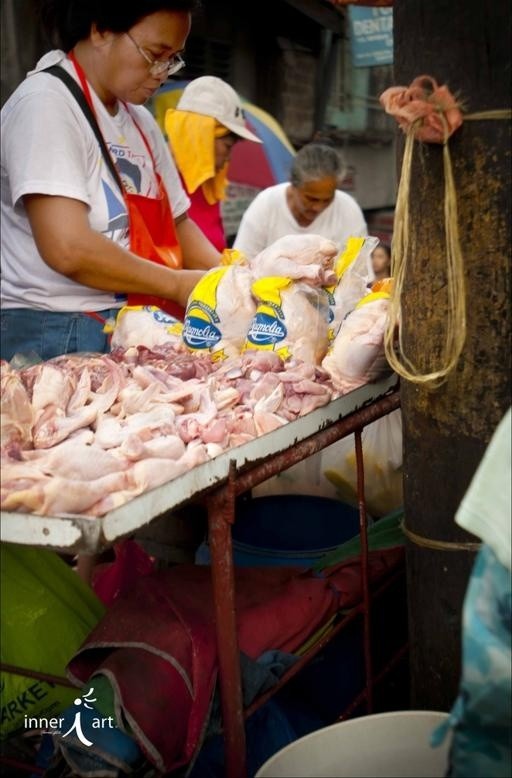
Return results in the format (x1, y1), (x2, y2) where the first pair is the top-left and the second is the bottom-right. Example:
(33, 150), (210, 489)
(0, 0), (227, 362)
(231, 144), (375, 287)
(429, 404), (512, 777)
(163, 75), (264, 256)
(370, 241), (392, 279)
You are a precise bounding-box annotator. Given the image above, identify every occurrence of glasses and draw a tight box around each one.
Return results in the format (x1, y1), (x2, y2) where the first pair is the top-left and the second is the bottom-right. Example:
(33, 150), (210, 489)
(125, 32), (187, 76)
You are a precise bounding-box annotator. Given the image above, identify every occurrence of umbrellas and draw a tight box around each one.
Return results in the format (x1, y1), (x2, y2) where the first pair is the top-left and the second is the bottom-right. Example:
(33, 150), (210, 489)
(152, 77), (296, 192)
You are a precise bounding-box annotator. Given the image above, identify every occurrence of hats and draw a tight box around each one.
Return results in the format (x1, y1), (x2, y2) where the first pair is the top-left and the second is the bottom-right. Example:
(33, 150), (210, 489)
(177, 74), (263, 145)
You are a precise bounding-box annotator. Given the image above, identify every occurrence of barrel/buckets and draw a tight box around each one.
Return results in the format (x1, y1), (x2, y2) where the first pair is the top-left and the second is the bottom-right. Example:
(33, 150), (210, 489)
(252, 709), (457, 778)
(194, 492), (377, 720)
